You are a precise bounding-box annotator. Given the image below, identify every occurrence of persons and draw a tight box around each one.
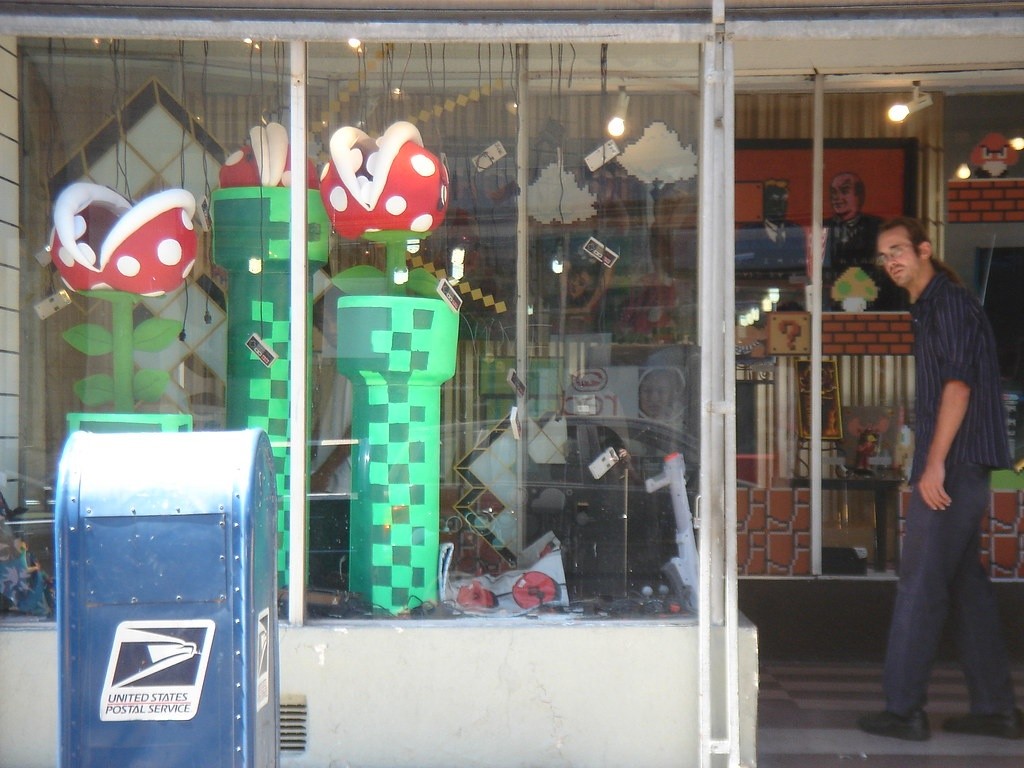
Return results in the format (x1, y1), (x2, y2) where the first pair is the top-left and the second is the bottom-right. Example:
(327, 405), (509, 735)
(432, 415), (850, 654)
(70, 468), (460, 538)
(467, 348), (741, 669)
(637, 367), (681, 419)
(857, 216), (1024, 739)
(310, 287), (353, 493)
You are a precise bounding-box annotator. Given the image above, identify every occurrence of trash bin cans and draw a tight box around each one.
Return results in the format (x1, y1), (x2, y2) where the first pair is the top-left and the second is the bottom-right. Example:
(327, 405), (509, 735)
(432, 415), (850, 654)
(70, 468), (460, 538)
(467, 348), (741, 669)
(54, 430), (280, 768)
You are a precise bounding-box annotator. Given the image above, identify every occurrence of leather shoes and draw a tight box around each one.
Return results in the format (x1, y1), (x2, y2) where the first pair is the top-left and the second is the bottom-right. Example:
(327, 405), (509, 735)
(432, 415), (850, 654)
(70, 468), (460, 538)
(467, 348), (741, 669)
(856, 709), (929, 741)
(944, 707), (1024, 738)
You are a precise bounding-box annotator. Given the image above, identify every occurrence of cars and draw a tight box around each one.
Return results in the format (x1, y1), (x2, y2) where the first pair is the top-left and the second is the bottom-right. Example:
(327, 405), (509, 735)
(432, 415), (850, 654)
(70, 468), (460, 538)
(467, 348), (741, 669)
(308, 413), (700, 597)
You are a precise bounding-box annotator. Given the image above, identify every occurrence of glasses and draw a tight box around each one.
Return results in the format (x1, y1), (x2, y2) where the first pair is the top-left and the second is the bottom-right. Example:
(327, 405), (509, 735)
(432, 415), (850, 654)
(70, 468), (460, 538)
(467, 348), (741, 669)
(874, 243), (914, 266)
